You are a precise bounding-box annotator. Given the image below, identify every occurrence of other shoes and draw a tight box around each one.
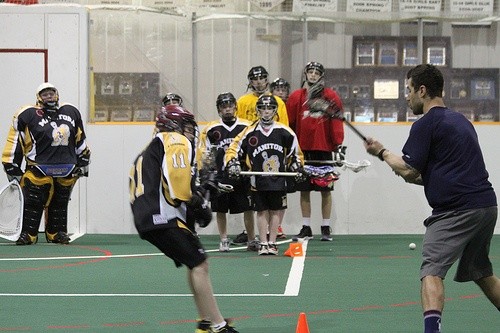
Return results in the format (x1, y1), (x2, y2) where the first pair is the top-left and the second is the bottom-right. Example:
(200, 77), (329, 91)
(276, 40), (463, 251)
(16, 232), (39, 246)
(194, 318), (211, 332)
(212, 321), (239, 333)
(45, 231), (72, 244)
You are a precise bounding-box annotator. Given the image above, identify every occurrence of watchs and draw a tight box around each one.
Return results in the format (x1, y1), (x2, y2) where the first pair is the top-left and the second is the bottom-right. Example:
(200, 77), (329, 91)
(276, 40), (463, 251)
(377, 148), (386, 161)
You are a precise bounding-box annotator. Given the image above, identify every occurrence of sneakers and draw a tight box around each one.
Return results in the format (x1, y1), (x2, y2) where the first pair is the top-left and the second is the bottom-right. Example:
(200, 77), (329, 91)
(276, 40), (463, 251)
(268, 242), (278, 254)
(292, 224), (314, 241)
(276, 229), (287, 239)
(319, 225), (333, 241)
(247, 241), (260, 251)
(219, 240), (230, 252)
(258, 243), (269, 255)
(230, 230), (248, 245)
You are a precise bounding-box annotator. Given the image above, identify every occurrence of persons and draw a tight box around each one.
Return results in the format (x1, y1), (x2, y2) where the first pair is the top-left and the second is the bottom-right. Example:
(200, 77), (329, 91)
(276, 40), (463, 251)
(230, 66), (289, 246)
(128, 105), (239, 333)
(363, 64), (500, 333)
(270, 77), (291, 236)
(196, 92), (261, 252)
(2, 82), (90, 246)
(152, 94), (199, 140)
(224, 94), (304, 255)
(285, 61), (344, 242)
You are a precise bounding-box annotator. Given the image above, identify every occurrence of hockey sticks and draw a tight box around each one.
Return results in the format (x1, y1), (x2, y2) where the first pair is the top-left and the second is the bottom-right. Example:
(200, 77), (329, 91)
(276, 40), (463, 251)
(239, 164), (335, 180)
(0, 177), (25, 242)
(303, 157), (371, 174)
(201, 144), (219, 206)
(306, 82), (371, 145)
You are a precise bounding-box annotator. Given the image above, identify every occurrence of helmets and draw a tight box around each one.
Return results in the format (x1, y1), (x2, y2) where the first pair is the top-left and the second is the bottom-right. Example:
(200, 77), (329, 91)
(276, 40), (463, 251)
(35, 83), (61, 110)
(254, 95), (280, 122)
(269, 78), (291, 92)
(216, 92), (238, 120)
(304, 61), (325, 82)
(247, 65), (272, 93)
(154, 105), (194, 134)
(161, 93), (185, 107)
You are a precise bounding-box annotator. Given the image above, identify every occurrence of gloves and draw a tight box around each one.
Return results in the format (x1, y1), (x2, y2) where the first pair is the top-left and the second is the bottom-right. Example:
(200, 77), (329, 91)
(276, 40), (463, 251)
(290, 162), (308, 184)
(333, 145), (348, 166)
(225, 157), (244, 181)
(188, 193), (213, 228)
(363, 136), (386, 162)
(215, 182), (235, 197)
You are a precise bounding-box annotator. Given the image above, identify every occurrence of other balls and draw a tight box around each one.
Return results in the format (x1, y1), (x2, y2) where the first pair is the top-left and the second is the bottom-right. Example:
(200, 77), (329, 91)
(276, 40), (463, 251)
(409, 243), (416, 249)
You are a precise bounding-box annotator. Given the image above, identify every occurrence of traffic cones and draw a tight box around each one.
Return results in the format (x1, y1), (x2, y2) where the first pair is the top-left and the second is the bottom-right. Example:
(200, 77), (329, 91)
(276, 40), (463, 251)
(296, 312), (311, 333)
